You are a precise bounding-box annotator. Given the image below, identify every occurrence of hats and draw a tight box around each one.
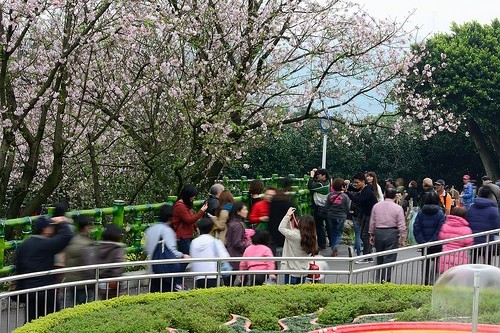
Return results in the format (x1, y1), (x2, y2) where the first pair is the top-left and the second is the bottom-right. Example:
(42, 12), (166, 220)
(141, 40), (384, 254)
(435, 179), (446, 186)
(36, 216), (58, 226)
(463, 174), (470, 179)
(78, 216), (95, 223)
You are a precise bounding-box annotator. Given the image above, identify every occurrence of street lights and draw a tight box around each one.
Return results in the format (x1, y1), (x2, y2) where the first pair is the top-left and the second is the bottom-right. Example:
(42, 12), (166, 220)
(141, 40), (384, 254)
(319, 106), (332, 170)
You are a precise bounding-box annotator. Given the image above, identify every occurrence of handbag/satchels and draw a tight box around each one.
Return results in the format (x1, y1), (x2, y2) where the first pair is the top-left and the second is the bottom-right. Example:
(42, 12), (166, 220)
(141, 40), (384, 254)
(307, 264), (320, 278)
(315, 204), (328, 217)
(217, 260), (233, 277)
(152, 236), (189, 285)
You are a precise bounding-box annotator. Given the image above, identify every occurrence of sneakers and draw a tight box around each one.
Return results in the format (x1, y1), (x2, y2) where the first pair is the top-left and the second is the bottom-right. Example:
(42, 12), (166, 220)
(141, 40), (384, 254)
(369, 258), (374, 262)
(354, 258), (369, 264)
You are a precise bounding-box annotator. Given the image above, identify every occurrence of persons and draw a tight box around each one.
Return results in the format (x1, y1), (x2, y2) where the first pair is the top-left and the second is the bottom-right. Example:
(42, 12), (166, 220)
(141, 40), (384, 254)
(9, 165), (499, 322)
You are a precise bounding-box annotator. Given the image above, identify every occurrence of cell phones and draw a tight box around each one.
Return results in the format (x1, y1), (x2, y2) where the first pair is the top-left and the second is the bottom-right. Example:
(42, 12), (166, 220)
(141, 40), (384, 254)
(290, 208), (296, 221)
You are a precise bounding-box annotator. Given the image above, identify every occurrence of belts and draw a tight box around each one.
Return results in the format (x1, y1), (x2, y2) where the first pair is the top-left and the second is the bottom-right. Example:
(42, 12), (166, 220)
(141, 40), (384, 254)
(374, 225), (398, 229)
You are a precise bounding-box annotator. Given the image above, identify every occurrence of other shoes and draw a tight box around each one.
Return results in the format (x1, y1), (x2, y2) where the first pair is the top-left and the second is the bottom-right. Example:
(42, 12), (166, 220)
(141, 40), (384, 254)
(331, 248), (338, 256)
